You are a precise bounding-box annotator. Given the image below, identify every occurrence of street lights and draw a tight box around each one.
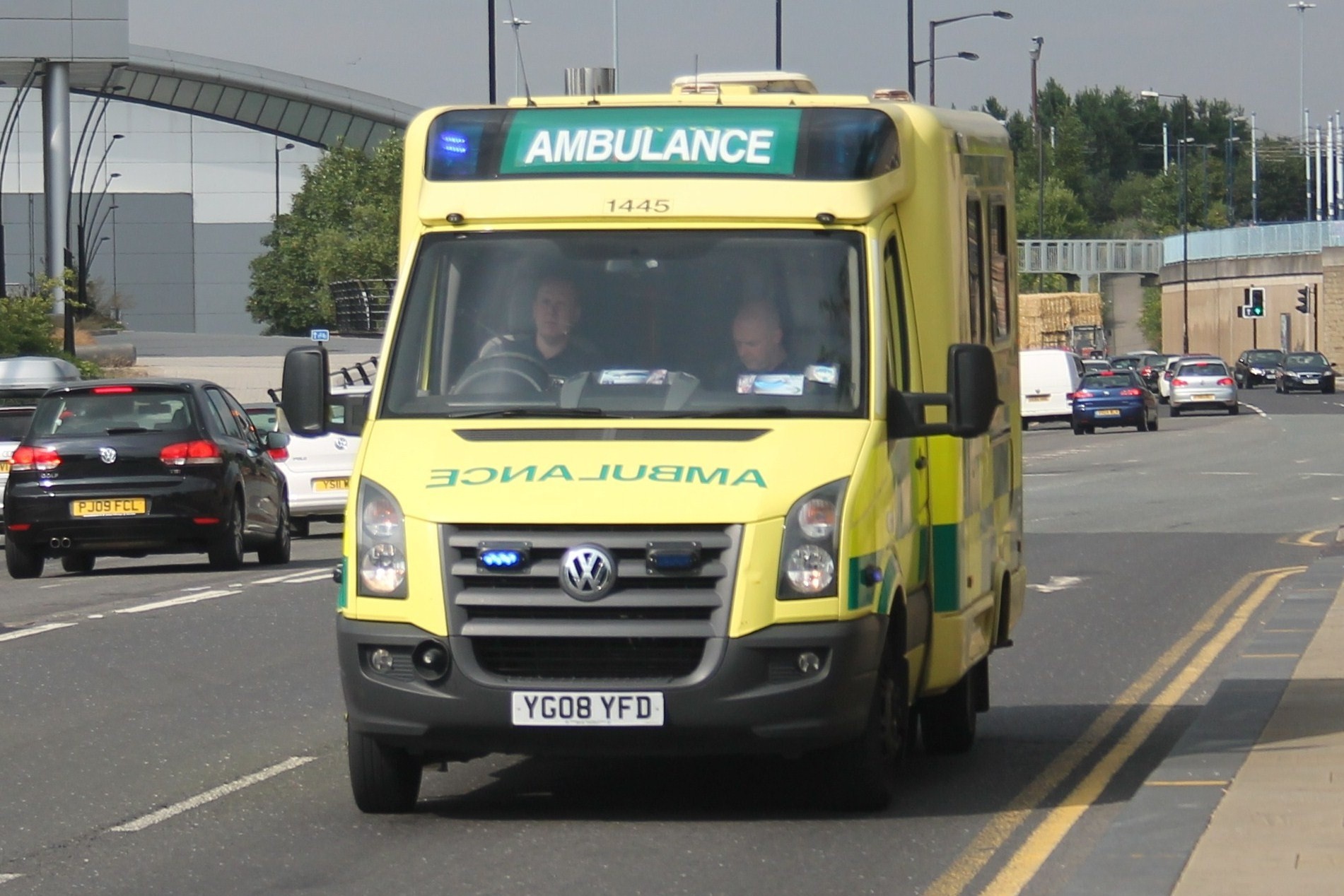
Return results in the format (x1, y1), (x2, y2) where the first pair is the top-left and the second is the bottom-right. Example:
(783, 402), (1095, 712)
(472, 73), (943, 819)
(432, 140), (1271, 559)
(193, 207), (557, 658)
(1177, 137), (1216, 230)
(0, 58), (45, 298)
(908, 0), (1014, 107)
(1225, 111), (1259, 226)
(1141, 92), (1189, 352)
(503, 18), (531, 96)
(276, 133), (295, 214)
(64, 61), (133, 355)
(1288, 1), (1317, 221)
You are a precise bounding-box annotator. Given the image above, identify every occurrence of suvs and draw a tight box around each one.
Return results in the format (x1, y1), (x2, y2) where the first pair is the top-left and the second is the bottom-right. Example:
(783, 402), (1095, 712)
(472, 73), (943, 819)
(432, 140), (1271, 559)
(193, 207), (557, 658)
(4, 378), (290, 578)
(1234, 349), (1283, 389)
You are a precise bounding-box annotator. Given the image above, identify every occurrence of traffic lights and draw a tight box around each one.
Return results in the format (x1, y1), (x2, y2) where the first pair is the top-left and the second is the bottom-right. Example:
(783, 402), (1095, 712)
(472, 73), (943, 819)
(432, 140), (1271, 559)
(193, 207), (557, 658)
(1242, 288), (1264, 318)
(1297, 286), (1310, 314)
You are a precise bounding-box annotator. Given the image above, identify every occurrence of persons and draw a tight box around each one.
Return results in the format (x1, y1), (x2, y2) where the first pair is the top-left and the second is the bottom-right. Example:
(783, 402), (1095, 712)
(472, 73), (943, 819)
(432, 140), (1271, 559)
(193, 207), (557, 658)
(477, 271), (824, 397)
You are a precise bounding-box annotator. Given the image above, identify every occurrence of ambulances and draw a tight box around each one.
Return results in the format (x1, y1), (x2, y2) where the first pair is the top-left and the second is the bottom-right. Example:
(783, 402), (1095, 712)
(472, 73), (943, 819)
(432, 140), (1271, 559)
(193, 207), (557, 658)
(282, 67), (1024, 814)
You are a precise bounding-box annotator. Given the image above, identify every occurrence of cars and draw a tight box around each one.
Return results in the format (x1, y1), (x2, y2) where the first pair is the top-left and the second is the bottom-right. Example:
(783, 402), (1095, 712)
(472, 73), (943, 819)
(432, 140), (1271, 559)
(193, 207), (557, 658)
(1072, 369), (1159, 434)
(1081, 350), (1220, 404)
(1168, 359), (1238, 417)
(1274, 352), (1337, 394)
(242, 386), (372, 538)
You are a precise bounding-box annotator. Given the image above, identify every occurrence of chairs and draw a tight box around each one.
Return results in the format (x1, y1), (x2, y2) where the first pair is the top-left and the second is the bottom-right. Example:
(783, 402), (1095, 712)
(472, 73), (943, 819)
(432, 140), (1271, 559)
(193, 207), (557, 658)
(508, 287), (539, 335)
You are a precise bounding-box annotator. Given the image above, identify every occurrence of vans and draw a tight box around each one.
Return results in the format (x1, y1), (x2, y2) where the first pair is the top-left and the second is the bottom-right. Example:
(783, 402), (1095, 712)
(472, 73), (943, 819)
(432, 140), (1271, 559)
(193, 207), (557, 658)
(1018, 350), (1086, 431)
(0, 356), (84, 532)
